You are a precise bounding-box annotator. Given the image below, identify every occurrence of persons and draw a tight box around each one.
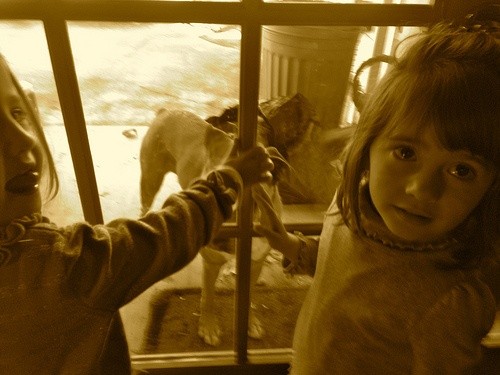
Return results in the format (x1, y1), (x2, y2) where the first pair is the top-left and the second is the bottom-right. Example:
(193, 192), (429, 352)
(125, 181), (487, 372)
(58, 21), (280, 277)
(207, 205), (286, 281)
(0, 51), (275, 375)
(253, 12), (500, 374)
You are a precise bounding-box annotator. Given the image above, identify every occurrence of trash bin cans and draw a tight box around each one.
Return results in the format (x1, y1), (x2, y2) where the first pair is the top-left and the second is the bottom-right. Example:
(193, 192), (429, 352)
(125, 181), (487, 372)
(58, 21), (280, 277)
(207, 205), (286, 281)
(245, 0), (372, 206)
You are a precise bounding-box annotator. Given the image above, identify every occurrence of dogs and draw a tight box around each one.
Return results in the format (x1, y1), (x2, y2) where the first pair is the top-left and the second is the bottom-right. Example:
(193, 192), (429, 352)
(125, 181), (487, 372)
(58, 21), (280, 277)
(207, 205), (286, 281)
(139, 91), (317, 347)
